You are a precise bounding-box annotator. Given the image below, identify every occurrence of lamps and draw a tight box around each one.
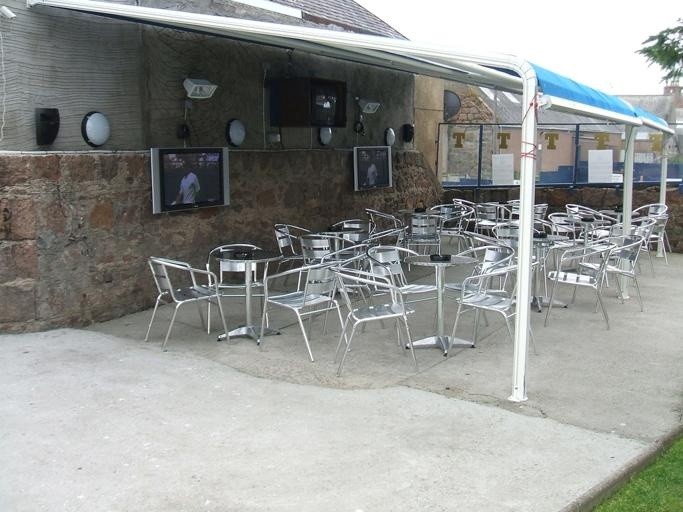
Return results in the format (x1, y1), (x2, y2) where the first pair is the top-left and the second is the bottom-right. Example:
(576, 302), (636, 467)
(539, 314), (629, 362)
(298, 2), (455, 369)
(184, 78), (218, 100)
(358, 97), (381, 115)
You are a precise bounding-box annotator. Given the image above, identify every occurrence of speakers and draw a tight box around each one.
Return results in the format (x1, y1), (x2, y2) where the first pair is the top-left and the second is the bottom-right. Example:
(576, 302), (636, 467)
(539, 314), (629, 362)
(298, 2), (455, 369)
(35, 107), (61, 146)
(403, 123), (415, 143)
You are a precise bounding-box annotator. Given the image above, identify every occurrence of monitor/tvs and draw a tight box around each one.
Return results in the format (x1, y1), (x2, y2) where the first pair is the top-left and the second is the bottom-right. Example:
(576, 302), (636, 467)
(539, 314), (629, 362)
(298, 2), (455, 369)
(353, 146), (392, 192)
(268, 75), (347, 128)
(150, 146), (231, 214)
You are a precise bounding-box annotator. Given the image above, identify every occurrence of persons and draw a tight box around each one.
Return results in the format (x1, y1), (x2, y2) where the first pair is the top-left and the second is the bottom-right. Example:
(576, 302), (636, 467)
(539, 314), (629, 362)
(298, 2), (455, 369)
(172, 162), (201, 205)
(364, 160), (382, 188)
